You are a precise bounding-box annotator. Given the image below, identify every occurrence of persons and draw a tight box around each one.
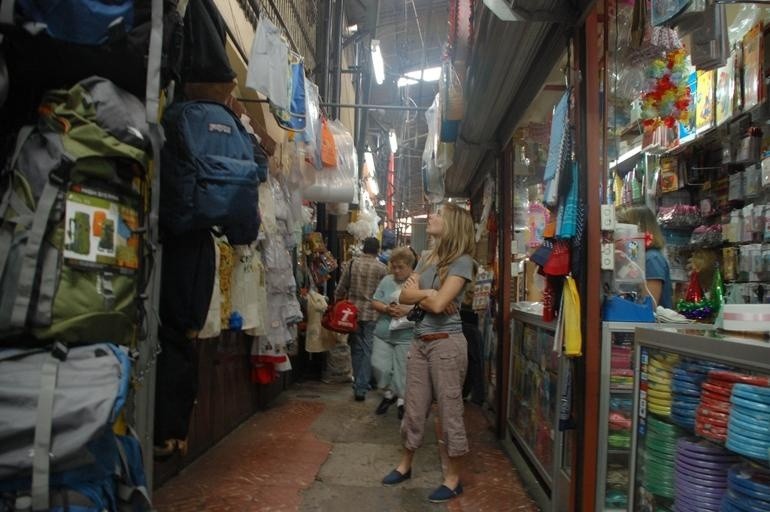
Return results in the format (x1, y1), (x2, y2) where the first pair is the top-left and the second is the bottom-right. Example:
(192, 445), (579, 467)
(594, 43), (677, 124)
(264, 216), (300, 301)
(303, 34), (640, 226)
(335, 235), (391, 401)
(370, 246), (416, 423)
(381, 200), (480, 503)
(616, 206), (673, 308)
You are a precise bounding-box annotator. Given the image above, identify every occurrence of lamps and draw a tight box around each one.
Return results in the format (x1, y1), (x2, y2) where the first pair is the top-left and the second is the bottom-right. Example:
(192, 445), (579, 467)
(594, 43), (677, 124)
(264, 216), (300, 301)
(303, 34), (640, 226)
(395, 66), (443, 86)
(389, 130), (398, 154)
(368, 35), (387, 85)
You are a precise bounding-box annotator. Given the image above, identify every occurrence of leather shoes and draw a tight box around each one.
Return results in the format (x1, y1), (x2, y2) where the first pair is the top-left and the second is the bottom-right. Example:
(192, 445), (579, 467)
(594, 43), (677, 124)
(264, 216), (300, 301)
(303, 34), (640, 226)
(382, 466), (411, 486)
(354, 394), (365, 403)
(397, 403), (406, 420)
(427, 480), (463, 502)
(374, 396), (399, 416)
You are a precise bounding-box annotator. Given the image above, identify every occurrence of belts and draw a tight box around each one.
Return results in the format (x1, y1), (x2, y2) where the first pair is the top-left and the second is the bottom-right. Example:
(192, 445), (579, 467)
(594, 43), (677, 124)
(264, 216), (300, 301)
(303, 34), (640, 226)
(418, 332), (452, 342)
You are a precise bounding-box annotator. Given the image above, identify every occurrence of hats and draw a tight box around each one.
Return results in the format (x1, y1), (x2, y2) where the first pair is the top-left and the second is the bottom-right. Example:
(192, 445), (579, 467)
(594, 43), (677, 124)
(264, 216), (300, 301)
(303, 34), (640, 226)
(361, 237), (381, 252)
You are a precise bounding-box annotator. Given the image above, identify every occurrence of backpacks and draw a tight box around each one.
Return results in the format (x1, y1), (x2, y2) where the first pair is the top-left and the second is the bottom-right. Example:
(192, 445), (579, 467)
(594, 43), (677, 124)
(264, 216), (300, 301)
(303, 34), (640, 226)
(1, 77), (153, 347)
(0, 345), (150, 512)
(9, 2), (174, 99)
(170, 106), (268, 243)
(178, 0), (243, 100)
